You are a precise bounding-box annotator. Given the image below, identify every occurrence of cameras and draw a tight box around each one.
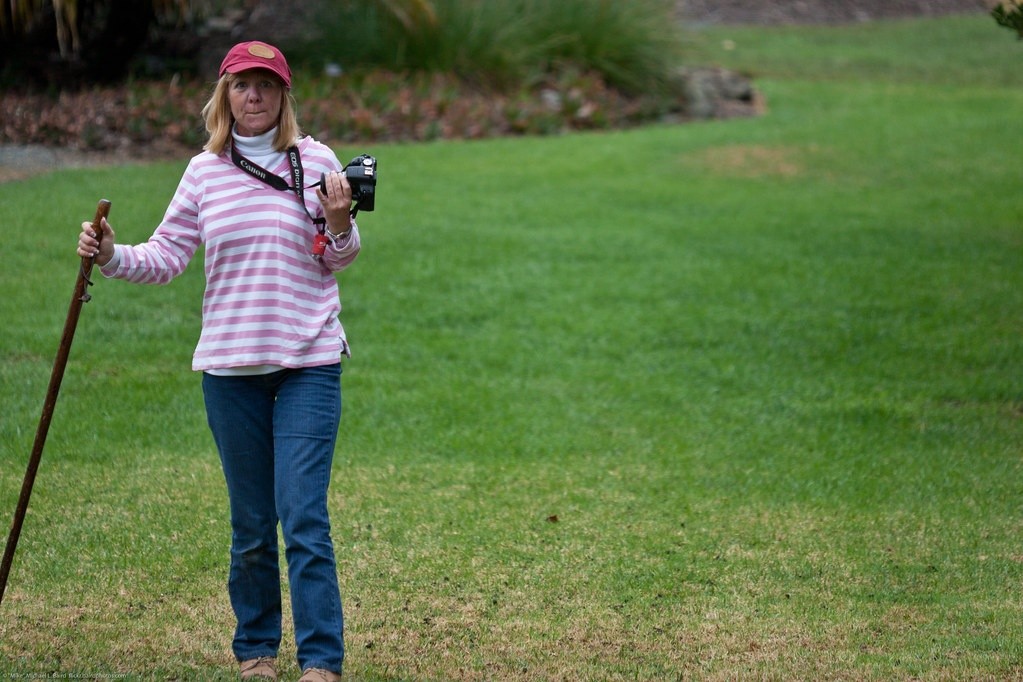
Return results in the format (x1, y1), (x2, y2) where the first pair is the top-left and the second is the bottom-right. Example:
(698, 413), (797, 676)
(320, 152), (377, 211)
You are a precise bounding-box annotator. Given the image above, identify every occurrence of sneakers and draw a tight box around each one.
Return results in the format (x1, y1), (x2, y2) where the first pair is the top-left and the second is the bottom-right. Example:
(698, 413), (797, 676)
(240, 656), (278, 681)
(300, 668), (341, 682)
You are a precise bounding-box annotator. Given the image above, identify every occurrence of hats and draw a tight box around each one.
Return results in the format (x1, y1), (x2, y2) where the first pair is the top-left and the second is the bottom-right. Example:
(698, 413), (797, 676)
(219, 40), (291, 91)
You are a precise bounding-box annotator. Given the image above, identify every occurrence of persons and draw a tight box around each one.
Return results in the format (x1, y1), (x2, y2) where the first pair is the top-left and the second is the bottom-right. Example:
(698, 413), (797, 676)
(74, 38), (362, 682)
